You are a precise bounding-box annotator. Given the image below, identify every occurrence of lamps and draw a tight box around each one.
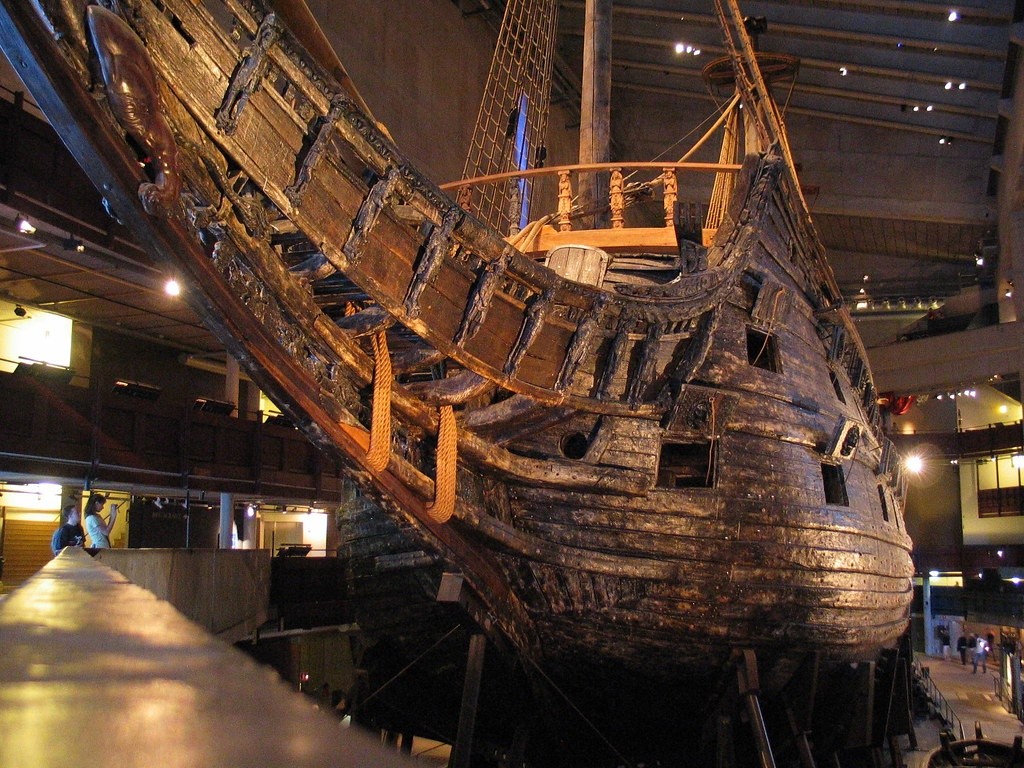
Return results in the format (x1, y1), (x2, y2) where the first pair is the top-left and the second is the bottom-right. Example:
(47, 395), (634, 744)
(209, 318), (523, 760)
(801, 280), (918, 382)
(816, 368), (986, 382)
(949, 393), (955, 399)
(957, 390), (962, 396)
(13, 212), (37, 234)
(180, 498), (190, 510)
(61, 232), (84, 253)
(932, 394), (942, 401)
(153, 497), (163, 510)
(248, 502), (260, 516)
(974, 246), (985, 266)
(198, 491), (205, 500)
(282, 506), (287, 514)
(1005, 289), (1011, 297)
(207, 498), (213, 510)
(991, 455), (996, 462)
(307, 509), (311, 515)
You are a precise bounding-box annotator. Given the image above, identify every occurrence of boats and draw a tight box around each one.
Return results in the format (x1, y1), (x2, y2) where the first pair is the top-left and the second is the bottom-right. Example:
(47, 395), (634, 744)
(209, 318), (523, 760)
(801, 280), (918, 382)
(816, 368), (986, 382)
(0, 0), (915, 740)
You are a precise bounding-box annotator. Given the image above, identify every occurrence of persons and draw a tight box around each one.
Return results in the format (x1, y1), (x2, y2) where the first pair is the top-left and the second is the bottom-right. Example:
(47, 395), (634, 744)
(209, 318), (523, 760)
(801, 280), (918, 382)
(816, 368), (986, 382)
(891, 423), (899, 434)
(50, 505), (85, 556)
(928, 308), (937, 320)
(957, 628), (996, 674)
(84, 494), (118, 548)
(940, 313), (945, 319)
(940, 629), (951, 662)
(321, 684), (334, 708)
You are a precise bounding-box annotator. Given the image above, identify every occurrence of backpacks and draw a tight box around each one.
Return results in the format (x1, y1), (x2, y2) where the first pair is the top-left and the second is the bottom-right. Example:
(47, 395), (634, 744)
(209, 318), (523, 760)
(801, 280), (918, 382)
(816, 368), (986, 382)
(51, 526), (65, 556)
